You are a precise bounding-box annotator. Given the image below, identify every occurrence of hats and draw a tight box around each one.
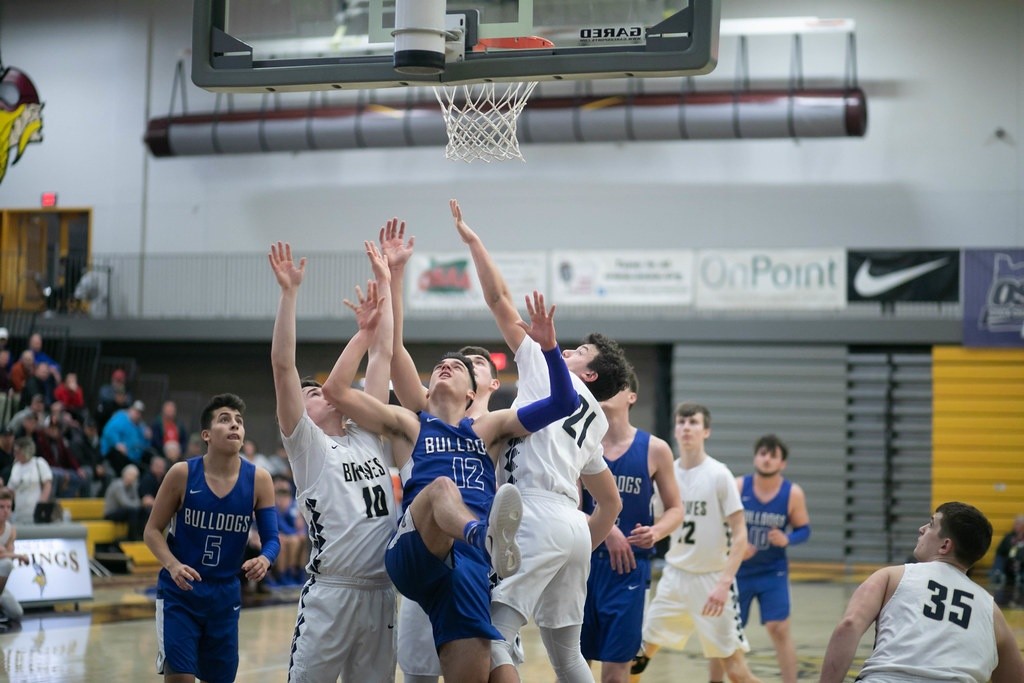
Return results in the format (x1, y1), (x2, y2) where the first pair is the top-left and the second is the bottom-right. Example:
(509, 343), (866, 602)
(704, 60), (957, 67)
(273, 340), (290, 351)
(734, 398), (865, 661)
(0, 326), (9, 340)
(113, 369), (125, 382)
(133, 400), (146, 413)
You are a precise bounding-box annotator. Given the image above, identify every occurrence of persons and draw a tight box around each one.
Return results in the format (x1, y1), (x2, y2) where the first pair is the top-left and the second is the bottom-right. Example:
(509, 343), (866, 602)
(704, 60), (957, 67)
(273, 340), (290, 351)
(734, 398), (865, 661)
(0, 330), (311, 631)
(381, 221), (528, 683)
(708, 434), (810, 683)
(322, 280), (581, 683)
(143, 394), (281, 683)
(630, 404), (765, 683)
(991, 517), (1024, 608)
(452, 199), (624, 683)
(821, 502), (1024, 683)
(270, 243), (420, 683)
(577, 372), (686, 683)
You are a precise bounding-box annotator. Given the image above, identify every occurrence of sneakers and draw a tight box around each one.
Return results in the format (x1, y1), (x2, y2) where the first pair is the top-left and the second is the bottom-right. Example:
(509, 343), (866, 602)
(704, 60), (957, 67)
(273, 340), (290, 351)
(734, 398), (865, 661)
(482, 483), (523, 578)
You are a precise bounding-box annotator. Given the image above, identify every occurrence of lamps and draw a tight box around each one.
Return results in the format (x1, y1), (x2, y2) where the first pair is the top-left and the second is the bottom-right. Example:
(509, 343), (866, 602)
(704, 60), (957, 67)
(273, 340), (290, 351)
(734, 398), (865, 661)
(392, 1), (449, 73)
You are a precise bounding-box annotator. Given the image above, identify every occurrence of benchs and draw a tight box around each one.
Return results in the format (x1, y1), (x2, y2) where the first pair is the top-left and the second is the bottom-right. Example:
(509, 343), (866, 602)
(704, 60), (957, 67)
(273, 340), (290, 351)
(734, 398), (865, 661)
(49, 494), (164, 569)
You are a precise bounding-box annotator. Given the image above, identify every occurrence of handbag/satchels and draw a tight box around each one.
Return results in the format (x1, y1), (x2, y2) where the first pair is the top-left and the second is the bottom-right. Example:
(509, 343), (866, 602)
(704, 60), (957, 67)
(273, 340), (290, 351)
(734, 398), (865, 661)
(33, 503), (56, 524)
